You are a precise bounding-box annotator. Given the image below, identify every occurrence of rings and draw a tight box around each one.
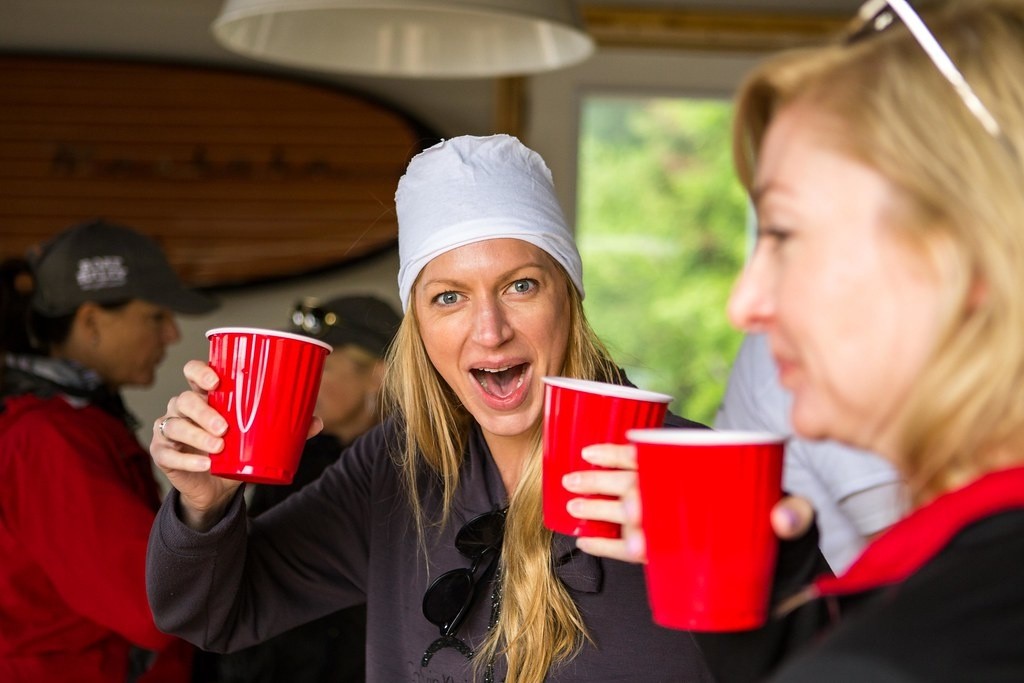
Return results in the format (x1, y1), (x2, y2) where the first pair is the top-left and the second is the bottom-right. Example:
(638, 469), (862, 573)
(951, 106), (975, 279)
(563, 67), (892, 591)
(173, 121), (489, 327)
(159, 416), (180, 437)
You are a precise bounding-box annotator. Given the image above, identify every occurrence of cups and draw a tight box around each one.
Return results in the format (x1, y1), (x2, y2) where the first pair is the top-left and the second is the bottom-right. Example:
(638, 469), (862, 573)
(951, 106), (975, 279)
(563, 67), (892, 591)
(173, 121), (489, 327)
(539, 373), (675, 539)
(204, 326), (334, 485)
(623, 428), (790, 632)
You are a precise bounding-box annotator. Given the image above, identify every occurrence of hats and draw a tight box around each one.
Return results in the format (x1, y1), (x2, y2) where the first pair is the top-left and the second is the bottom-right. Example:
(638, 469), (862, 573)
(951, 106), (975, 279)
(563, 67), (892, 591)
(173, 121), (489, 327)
(294, 293), (402, 356)
(37, 221), (219, 315)
(394, 133), (585, 316)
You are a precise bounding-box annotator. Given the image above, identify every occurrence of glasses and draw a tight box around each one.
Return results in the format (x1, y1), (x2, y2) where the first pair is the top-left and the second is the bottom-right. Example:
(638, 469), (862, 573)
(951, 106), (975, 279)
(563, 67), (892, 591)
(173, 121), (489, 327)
(847, 0), (1000, 137)
(422, 504), (509, 641)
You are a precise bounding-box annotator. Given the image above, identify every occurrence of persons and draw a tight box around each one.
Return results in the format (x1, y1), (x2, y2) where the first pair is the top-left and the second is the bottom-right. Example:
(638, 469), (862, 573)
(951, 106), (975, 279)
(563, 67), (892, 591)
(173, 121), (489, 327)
(0, 215), (185, 683)
(725, 0), (1024, 682)
(151, 133), (837, 683)
